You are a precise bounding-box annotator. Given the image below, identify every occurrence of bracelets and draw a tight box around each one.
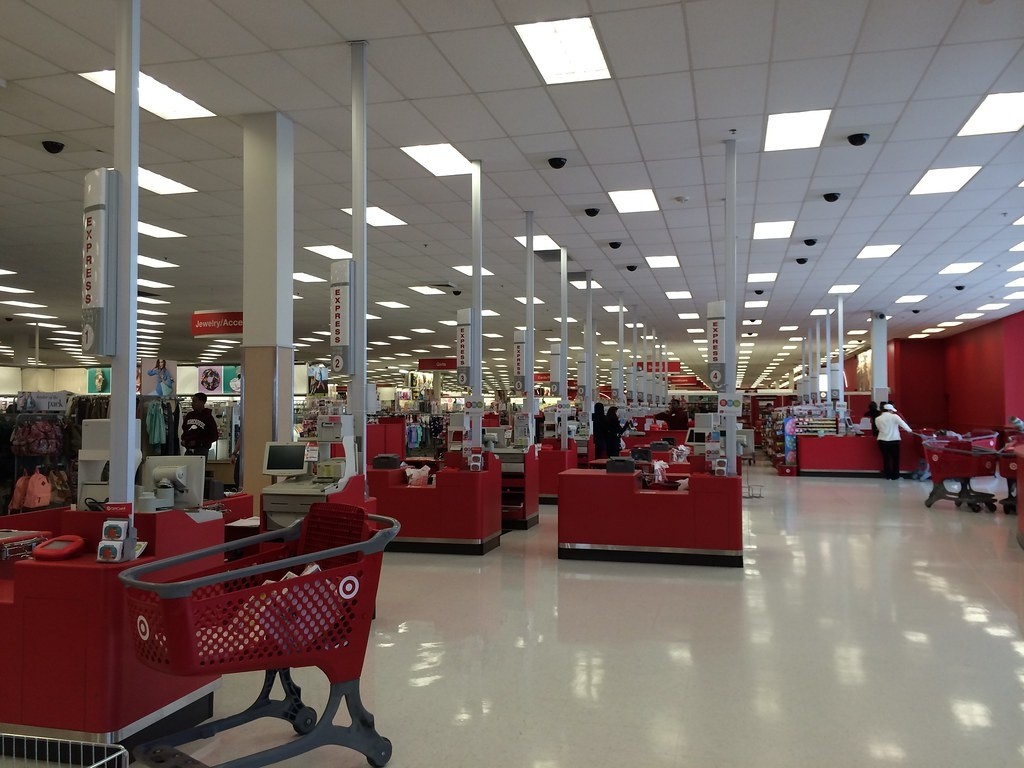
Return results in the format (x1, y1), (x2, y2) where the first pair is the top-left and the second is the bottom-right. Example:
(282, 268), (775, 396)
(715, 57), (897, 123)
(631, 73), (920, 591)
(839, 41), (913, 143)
(232, 452), (237, 457)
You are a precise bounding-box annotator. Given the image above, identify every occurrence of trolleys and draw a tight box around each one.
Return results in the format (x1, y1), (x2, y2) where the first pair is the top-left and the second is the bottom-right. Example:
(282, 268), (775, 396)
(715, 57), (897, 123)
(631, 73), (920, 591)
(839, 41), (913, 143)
(111, 501), (396, 768)
(999, 423), (1024, 514)
(922, 437), (998, 513)
(910, 427), (997, 488)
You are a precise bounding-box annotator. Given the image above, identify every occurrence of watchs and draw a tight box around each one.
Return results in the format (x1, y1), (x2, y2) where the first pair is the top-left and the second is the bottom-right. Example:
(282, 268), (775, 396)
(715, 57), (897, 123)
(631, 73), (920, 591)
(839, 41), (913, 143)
(95, 368), (105, 392)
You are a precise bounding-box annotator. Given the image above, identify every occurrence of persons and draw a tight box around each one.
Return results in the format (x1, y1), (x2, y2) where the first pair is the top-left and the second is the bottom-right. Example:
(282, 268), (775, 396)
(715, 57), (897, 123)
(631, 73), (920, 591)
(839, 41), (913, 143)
(181, 392), (218, 466)
(147, 359), (175, 395)
(655, 399), (689, 430)
(230, 437), (241, 492)
(605, 406), (632, 457)
(874, 404), (913, 480)
(864, 401), (907, 437)
(763, 403), (774, 414)
(592, 403), (606, 459)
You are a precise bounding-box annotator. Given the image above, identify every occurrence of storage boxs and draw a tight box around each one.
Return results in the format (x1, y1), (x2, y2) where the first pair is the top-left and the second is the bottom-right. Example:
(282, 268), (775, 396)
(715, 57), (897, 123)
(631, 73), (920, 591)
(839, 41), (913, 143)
(450, 414), (470, 426)
(516, 412), (532, 427)
(317, 415), (354, 442)
(83, 419), (140, 451)
(545, 412), (556, 421)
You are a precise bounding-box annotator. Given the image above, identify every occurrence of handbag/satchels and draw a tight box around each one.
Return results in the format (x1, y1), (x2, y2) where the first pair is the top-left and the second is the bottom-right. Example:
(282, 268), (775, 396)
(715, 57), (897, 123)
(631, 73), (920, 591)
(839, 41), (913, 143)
(160, 382), (174, 397)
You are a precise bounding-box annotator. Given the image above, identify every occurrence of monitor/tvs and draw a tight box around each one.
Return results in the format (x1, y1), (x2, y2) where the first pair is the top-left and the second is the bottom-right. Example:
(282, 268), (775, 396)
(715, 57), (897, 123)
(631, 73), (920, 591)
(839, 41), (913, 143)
(685, 428), (707, 445)
(487, 427), (505, 448)
(451, 429), (463, 442)
(144, 455), (205, 508)
(263, 442), (309, 476)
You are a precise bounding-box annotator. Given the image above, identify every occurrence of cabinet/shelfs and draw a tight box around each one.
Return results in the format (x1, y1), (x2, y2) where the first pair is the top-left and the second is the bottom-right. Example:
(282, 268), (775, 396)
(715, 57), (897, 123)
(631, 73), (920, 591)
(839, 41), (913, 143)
(760, 412), (797, 476)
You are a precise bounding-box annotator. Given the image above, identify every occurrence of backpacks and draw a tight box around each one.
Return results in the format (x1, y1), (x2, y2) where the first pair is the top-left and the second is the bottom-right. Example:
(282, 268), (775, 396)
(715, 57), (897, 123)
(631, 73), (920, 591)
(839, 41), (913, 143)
(6, 394), (37, 415)
(10, 418), (63, 458)
(8, 467), (72, 510)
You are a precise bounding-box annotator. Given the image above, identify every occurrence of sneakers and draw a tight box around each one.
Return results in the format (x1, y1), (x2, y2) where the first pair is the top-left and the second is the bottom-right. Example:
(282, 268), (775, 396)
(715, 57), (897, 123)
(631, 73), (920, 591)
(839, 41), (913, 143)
(886, 476), (905, 480)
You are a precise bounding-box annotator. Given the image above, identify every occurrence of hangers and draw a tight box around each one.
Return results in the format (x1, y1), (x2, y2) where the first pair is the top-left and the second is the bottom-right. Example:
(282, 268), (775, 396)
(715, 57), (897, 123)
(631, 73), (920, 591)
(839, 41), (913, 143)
(72, 394), (181, 407)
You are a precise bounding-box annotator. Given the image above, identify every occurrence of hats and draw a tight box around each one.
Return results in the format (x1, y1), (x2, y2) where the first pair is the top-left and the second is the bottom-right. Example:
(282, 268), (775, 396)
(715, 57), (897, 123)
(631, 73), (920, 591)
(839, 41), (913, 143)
(883, 404), (897, 412)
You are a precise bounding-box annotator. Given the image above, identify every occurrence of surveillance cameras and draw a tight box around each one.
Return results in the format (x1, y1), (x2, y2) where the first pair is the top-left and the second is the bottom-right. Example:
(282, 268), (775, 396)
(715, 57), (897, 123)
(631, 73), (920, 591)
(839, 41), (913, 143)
(877, 313), (885, 319)
(913, 310), (919, 313)
(453, 291), (462, 295)
(804, 239), (818, 246)
(956, 286), (965, 290)
(748, 333), (752, 335)
(42, 141), (65, 154)
(848, 133), (870, 146)
(750, 319), (755, 322)
(755, 290), (764, 295)
(626, 266), (637, 271)
(796, 258), (809, 264)
(585, 208), (600, 217)
(548, 158), (567, 169)
(823, 193), (840, 202)
(843, 348), (846, 350)
(858, 340), (862, 342)
(609, 242), (621, 249)
